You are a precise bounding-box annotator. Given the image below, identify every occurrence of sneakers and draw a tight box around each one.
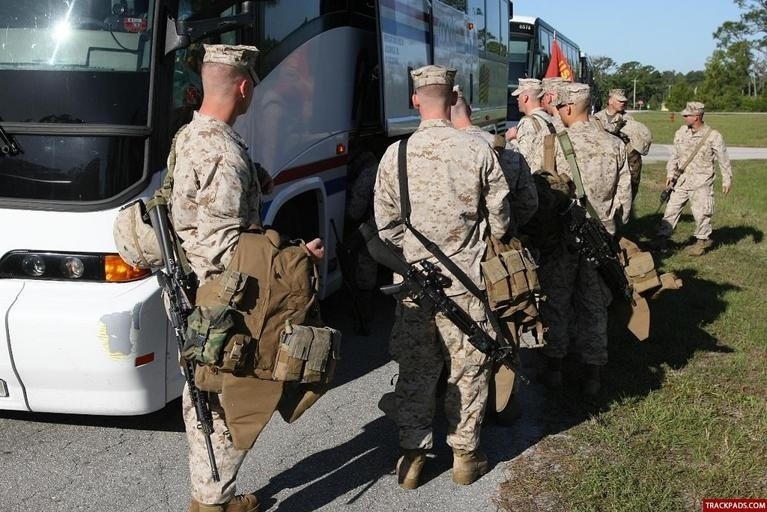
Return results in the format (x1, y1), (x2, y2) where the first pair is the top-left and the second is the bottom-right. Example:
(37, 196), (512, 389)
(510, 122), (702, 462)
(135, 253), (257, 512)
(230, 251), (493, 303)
(689, 240), (705, 255)
(189, 494), (261, 512)
(540, 368), (562, 388)
(396, 451), (426, 490)
(452, 449), (488, 485)
(584, 381), (601, 395)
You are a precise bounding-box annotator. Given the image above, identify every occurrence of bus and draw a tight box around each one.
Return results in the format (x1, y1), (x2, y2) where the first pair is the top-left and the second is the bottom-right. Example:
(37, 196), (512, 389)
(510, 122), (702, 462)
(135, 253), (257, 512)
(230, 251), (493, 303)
(508, 17), (587, 122)
(0, 1), (511, 416)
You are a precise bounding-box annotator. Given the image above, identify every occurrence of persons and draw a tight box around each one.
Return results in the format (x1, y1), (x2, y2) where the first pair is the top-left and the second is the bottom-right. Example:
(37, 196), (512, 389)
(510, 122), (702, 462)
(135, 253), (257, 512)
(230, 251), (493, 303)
(170, 42), (326, 512)
(432, 78), (655, 428)
(654, 101), (733, 257)
(373, 63), (511, 490)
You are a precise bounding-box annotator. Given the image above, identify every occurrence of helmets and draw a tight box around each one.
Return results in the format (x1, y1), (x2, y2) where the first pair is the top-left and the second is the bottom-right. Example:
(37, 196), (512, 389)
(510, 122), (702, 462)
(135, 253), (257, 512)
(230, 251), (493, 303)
(620, 120), (653, 155)
(113, 200), (164, 270)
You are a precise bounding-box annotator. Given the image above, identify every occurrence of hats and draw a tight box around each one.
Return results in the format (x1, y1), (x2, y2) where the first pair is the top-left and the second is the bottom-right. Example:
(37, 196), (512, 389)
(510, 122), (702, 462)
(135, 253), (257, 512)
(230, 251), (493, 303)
(410, 66), (456, 93)
(202, 43), (261, 84)
(511, 77), (590, 106)
(609, 89), (628, 102)
(680, 102), (705, 115)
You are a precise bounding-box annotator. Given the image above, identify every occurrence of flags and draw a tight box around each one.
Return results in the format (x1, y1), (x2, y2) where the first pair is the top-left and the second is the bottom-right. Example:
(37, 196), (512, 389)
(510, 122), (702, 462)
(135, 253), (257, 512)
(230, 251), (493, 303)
(543, 39), (578, 83)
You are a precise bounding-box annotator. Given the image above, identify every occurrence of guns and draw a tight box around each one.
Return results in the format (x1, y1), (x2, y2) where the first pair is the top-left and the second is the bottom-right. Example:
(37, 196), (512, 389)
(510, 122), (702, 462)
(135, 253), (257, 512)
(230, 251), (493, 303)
(146, 197), (219, 484)
(654, 179), (677, 213)
(568, 207), (637, 307)
(357, 223), (531, 385)
